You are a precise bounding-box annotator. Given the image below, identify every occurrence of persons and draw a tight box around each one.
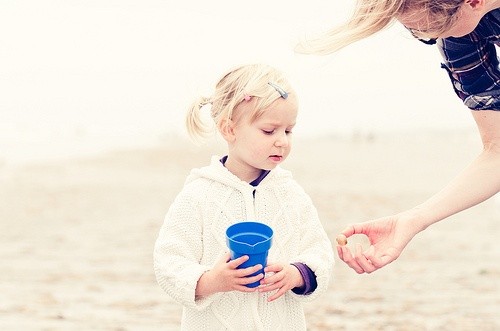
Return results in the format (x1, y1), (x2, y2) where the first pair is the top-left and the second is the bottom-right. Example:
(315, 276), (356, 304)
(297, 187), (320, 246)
(151, 63), (335, 331)
(289, 1), (500, 274)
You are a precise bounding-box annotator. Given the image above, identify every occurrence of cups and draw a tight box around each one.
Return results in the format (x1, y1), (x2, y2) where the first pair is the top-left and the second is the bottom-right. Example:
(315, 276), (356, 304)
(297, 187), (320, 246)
(225, 221), (274, 288)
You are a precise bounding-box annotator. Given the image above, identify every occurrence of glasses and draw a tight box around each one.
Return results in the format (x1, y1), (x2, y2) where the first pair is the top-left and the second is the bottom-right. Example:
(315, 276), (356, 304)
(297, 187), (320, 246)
(404, 0), (464, 45)
